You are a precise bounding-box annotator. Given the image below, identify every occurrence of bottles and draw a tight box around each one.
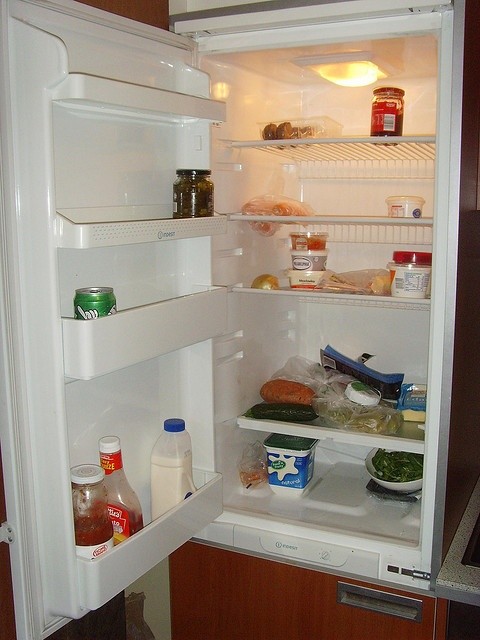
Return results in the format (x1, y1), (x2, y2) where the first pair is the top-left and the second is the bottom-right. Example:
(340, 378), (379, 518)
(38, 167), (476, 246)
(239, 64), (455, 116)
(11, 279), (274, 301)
(96, 436), (145, 549)
(69, 463), (115, 559)
(369, 86), (405, 147)
(149, 418), (194, 522)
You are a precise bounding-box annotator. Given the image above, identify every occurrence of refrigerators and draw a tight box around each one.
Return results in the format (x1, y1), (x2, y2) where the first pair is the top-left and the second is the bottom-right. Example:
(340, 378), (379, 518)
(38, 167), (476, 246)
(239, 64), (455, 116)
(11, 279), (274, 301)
(0, 0), (480, 640)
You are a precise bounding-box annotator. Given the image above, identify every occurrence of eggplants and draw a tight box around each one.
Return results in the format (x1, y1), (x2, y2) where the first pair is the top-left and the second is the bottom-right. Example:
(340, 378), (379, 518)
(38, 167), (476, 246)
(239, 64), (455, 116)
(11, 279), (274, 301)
(242, 401), (320, 422)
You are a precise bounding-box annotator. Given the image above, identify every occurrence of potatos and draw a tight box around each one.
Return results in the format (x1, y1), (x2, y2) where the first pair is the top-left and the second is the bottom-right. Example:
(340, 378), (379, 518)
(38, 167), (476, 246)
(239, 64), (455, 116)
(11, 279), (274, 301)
(261, 378), (316, 407)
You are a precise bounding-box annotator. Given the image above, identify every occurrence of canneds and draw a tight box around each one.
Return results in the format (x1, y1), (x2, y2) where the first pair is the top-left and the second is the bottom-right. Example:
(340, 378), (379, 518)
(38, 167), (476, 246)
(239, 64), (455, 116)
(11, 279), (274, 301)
(74, 286), (118, 320)
(370, 86), (406, 137)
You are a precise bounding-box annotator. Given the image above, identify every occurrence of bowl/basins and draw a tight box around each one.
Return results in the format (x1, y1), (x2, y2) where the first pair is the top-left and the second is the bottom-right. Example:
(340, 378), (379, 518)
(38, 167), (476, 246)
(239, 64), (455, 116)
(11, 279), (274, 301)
(365, 447), (423, 495)
